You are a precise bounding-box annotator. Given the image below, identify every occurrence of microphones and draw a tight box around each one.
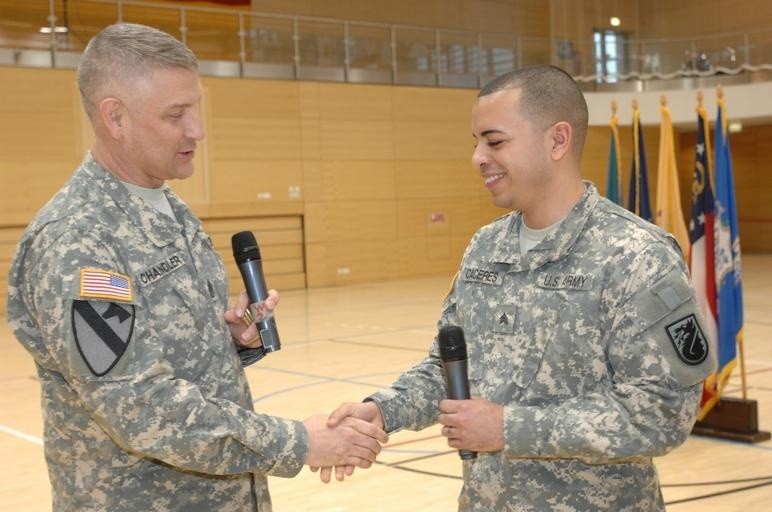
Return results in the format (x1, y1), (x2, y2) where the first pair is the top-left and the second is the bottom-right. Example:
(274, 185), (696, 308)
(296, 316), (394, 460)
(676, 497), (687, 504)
(438, 326), (478, 460)
(231, 232), (281, 352)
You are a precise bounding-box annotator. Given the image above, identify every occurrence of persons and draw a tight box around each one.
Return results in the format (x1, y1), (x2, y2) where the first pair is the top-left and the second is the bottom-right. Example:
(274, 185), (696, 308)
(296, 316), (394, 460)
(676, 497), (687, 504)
(5, 20), (391, 512)
(310, 63), (719, 512)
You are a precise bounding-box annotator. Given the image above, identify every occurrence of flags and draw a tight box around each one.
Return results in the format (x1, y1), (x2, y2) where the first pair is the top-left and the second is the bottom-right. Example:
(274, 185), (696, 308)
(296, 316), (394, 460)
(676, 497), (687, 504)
(627, 109), (652, 223)
(685, 104), (732, 422)
(654, 103), (692, 265)
(605, 112), (623, 205)
(710, 98), (745, 388)
